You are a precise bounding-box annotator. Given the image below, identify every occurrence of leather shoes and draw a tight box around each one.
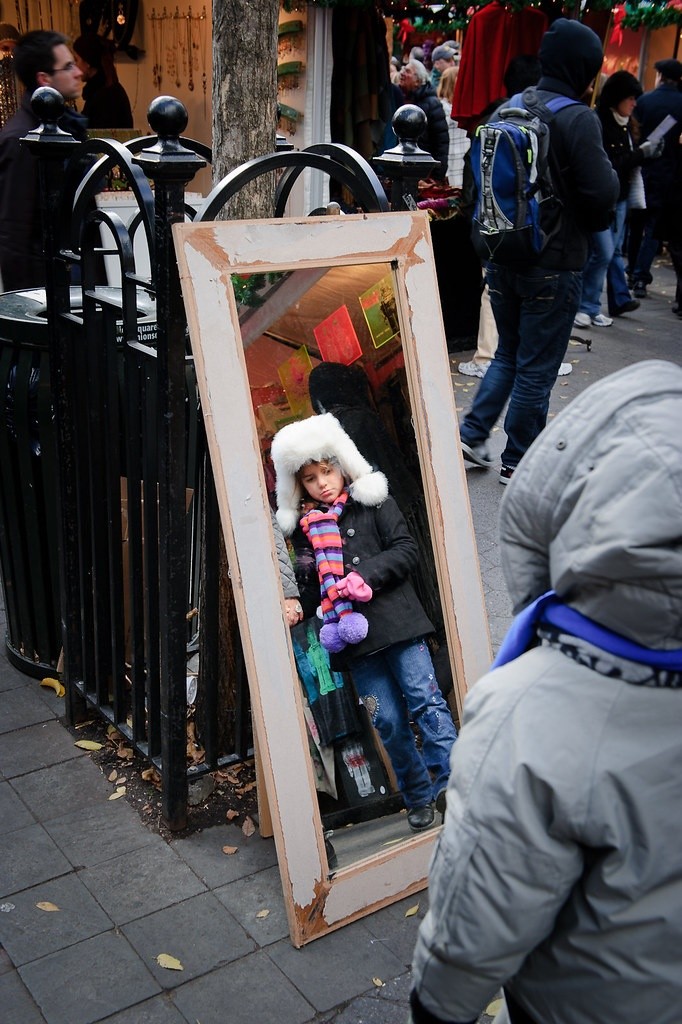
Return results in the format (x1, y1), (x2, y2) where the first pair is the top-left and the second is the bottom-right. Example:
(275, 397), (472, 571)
(407, 801), (436, 834)
(324, 837), (338, 868)
(436, 790), (447, 824)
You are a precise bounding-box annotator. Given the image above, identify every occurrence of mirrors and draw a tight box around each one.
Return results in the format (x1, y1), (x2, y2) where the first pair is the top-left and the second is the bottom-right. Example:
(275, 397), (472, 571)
(170, 212), (493, 947)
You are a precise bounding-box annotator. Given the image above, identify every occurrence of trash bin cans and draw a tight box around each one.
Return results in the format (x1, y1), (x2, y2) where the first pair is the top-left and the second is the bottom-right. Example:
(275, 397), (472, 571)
(431, 207), (484, 353)
(0, 286), (156, 681)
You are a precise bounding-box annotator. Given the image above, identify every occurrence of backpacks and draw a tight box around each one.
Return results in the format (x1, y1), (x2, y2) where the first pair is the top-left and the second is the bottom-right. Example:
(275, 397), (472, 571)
(471, 95), (585, 270)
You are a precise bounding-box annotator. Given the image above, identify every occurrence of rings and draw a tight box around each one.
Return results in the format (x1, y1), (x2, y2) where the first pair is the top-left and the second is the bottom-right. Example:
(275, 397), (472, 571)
(295, 603), (303, 613)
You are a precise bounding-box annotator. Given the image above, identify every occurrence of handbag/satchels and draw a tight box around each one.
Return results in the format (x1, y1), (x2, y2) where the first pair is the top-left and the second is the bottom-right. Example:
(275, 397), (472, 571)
(626, 130), (647, 210)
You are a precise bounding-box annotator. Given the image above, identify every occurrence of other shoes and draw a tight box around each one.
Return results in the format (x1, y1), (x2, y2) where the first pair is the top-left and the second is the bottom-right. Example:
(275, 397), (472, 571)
(672, 305), (682, 319)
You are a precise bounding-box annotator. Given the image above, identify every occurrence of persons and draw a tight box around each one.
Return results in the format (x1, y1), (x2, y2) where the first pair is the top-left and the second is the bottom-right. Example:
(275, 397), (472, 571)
(388, 40), (469, 189)
(461, 20), (624, 487)
(0, 30), (83, 140)
(71, 33), (135, 128)
(272, 514), (340, 870)
(460, 54), (682, 376)
(273, 410), (461, 833)
(410, 358), (682, 1024)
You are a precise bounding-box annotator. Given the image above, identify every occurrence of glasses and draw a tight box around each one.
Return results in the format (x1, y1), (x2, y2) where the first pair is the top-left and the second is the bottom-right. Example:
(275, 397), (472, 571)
(44, 61), (79, 73)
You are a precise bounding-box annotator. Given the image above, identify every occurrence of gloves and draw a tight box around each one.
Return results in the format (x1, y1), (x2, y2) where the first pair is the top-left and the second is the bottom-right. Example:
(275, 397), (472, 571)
(639, 139), (661, 158)
(653, 137), (665, 159)
(336, 564), (372, 603)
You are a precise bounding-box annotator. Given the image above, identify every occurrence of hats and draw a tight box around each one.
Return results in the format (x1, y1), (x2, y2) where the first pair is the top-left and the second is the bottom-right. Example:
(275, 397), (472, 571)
(73, 33), (104, 69)
(269, 413), (389, 539)
(431, 45), (459, 60)
(655, 59), (682, 82)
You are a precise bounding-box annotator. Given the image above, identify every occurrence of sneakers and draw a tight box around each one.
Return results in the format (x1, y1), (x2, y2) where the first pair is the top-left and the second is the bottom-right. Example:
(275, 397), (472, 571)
(633, 280), (647, 298)
(558, 362), (572, 375)
(458, 433), (492, 468)
(591, 314), (613, 327)
(611, 299), (641, 315)
(499, 464), (517, 485)
(626, 277), (634, 289)
(458, 359), (491, 378)
(574, 312), (591, 328)
